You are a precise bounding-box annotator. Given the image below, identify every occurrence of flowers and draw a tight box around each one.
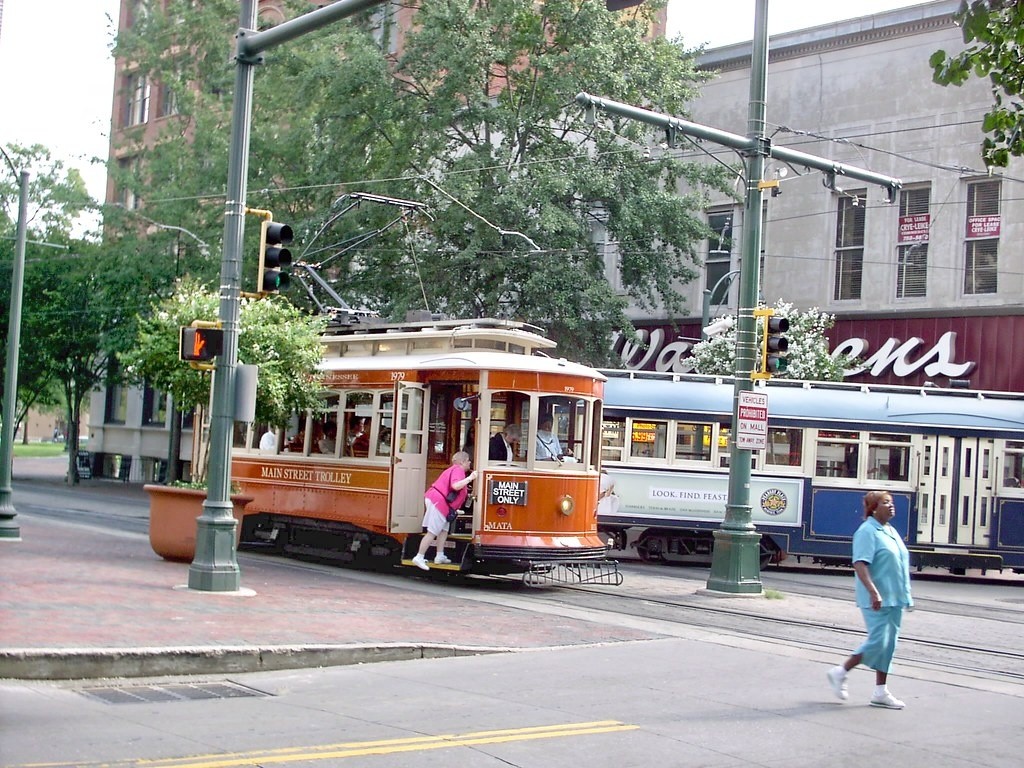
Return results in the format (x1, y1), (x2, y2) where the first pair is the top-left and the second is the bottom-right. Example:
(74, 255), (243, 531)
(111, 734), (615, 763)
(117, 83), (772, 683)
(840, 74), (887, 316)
(114, 273), (337, 493)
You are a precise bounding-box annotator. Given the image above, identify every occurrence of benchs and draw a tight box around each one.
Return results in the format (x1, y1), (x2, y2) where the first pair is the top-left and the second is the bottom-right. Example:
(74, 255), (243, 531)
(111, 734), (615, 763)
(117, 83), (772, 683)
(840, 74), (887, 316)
(285, 443), (321, 453)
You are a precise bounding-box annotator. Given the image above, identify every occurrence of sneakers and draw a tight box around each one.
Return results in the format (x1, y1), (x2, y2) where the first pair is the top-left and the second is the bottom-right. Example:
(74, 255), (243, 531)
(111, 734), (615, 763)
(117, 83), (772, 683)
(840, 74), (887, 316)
(872, 695), (903, 710)
(413, 556), (430, 570)
(826, 671), (849, 700)
(435, 555), (452, 566)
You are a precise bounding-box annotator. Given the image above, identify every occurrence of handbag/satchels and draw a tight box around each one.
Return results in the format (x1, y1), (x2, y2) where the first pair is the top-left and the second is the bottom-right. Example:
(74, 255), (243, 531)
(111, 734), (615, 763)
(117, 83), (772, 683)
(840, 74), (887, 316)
(448, 508), (457, 522)
(446, 491), (459, 503)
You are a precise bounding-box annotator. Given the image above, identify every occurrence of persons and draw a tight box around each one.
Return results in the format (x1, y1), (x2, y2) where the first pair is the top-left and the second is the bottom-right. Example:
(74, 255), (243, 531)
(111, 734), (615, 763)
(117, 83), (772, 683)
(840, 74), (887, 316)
(462, 425), (475, 468)
(535, 413), (575, 463)
(54, 426), (68, 442)
(412, 451), (478, 570)
(259, 414), (407, 458)
(826, 491), (915, 711)
(489, 424), (522, 461)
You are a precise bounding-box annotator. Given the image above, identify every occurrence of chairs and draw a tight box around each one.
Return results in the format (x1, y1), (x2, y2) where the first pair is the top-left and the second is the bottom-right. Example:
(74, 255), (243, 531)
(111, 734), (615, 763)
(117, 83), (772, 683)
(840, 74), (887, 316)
(351, 436), (369, 457)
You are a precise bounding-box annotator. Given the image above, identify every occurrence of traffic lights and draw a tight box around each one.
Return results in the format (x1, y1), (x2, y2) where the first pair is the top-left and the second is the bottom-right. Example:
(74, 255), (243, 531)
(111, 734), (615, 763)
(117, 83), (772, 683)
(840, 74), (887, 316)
(762, 312), (790, 375)
(179, 325), (217, 362)
(258, 220), (293, 294)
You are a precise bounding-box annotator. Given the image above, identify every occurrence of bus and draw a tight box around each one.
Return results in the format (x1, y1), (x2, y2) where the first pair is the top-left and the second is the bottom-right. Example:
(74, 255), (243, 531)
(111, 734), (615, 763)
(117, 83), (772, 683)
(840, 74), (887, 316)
(537, 369), (1024, 576)
(188, 306), (623, 590)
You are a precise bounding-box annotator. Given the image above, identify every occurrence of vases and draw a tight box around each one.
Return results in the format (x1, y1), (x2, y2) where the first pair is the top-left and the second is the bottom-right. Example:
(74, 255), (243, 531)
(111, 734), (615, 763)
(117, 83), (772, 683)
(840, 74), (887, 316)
(142, 484), (255, 563)
